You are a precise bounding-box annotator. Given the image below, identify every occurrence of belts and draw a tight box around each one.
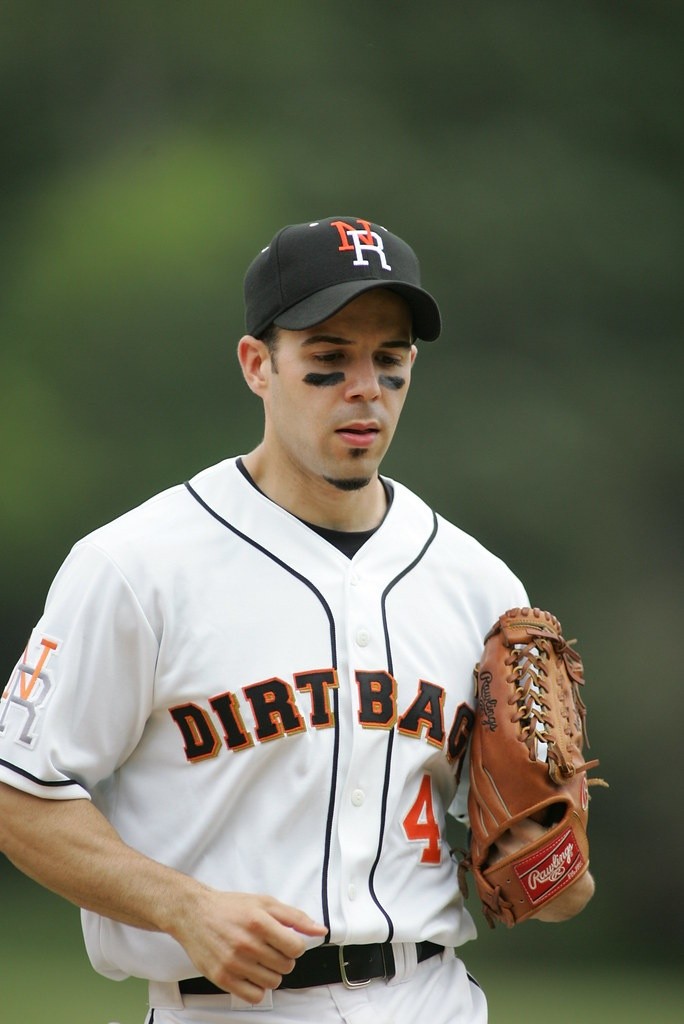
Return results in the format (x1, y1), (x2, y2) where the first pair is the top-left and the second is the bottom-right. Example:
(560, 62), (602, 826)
(179, 938), (447, 994)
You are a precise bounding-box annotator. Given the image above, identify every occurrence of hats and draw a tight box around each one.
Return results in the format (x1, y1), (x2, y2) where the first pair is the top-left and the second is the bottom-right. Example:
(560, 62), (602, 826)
(245, 213), (443, 344)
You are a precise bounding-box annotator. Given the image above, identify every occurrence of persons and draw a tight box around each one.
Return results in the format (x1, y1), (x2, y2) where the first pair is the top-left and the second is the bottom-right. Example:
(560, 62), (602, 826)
(0, 214), (611, 1024)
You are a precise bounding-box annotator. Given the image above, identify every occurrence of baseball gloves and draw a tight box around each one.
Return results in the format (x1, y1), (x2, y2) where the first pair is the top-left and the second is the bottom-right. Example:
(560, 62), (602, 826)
(466, 605), (595, 931)
